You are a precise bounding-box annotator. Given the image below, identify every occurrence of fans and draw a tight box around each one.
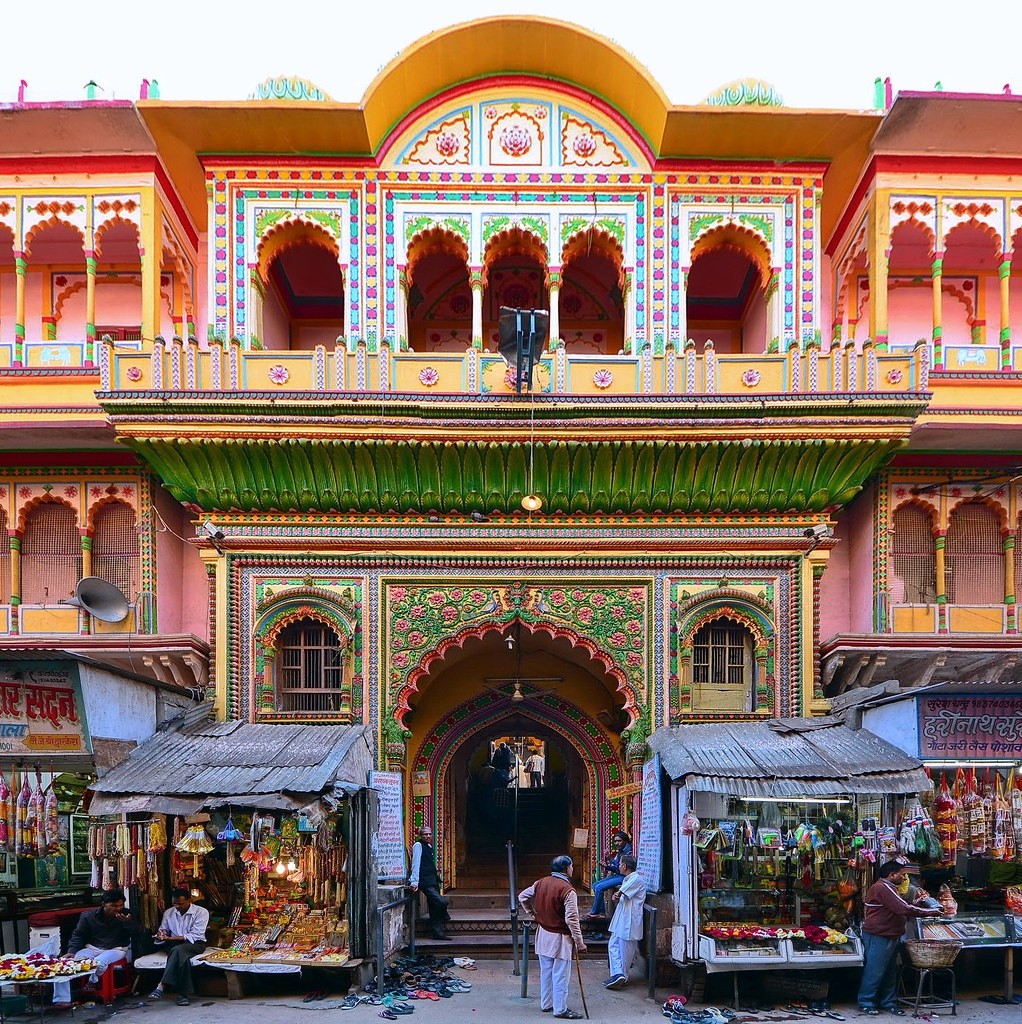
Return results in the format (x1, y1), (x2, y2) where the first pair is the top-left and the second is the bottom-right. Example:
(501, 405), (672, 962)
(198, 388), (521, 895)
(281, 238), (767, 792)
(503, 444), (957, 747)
(481, 654), (558, 702)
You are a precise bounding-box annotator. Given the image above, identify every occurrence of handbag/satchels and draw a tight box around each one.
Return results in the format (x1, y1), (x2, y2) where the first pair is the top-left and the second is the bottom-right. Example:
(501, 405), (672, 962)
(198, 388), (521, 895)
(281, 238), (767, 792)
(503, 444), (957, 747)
(523, 762), (534, 773)
(1005, 886), (1022, 917)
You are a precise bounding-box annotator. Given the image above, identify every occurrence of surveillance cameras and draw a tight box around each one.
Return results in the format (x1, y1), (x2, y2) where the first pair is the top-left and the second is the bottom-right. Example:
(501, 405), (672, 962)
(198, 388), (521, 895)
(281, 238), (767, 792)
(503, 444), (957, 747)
(203, 520), (225, 541)
(803, 524), (828, 540)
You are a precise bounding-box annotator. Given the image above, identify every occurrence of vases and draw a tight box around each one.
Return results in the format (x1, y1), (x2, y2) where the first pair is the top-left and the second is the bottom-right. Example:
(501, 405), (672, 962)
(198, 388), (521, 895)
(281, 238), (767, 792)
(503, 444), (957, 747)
(921, 342), (926, 346)
(102, 339), (111, 344)
(846, 343), (855, 350)
(863, 344), (873, 349)
(557, 343), (564, 348)
(705, 345), (713, 350)
(687, 345), (695, 350)
(337, 342), (345, 347)
(789, 343), (798, 350)
(830, 344), (841, 350)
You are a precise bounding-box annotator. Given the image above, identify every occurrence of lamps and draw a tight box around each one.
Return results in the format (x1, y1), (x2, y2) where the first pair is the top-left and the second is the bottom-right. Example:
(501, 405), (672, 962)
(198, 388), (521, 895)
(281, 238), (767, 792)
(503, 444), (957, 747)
(520, 378), (541, 512)
(504, 633), (517, 650)
(597, 710), (616, 727)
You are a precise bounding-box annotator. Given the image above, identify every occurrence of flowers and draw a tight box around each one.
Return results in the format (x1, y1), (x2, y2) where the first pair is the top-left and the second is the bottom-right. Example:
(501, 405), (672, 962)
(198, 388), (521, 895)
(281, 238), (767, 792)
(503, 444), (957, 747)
(844, 339), (856, 346)
(862, 338), (872, 346)
(830, 338), (839, 346)
(704, 339), (713, 347)
(916, 337), (927, 345)
(102, 333), (112, 341)
(685, 338), (696, 346)
(557, 338), (565, 345)
(788, 338), (799, 346)
(336, 335), (346, 343)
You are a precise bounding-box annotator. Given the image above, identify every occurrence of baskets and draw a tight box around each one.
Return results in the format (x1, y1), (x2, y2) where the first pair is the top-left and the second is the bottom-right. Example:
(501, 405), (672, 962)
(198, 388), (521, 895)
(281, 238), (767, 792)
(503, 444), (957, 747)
(905, 938), (963, 967)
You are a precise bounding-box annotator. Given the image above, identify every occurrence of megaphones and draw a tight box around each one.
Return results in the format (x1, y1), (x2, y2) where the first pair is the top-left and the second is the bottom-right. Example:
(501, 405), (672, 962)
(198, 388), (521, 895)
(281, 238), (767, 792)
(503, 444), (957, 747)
(62, 575), (129, 622)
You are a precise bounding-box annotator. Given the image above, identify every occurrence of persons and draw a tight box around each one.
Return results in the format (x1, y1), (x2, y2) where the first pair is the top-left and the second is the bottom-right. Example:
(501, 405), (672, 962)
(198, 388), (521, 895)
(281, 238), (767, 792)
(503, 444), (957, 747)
(60, 889), (133, 991)
(410, 826), (453, 940)
(585, 831), (647, 990)
(477, 742), (544, 840)
(518, 855), (587, 1018)
(858, 860), (945, 1016)
(147, 888), (209, 1006)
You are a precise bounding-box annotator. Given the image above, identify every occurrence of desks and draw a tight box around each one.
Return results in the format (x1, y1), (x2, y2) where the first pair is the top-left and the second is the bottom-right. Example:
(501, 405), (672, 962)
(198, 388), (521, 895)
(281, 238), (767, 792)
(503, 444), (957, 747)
(0, 966), (99, 1024)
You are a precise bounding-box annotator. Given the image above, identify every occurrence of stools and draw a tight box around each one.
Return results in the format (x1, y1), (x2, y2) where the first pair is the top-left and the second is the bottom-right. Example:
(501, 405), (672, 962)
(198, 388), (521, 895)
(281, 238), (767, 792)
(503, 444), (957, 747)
(74, 957), (132, 1006)
(895, 961), (960, 1018)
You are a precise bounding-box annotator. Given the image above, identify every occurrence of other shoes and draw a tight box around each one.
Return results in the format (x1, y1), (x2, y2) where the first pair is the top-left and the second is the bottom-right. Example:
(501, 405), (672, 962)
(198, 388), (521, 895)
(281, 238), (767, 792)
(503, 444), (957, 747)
(303, 988), (331, 1002)
(554, 1008), (583, 1019)
(431, 923), (452, 940)
(585, 912), (606, 918)
(540, 1005), (571, 1012)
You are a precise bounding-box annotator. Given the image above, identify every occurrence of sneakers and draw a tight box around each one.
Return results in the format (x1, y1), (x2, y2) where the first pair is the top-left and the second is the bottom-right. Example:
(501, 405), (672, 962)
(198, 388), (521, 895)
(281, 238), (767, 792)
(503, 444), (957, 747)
(601, 974), (629, 989)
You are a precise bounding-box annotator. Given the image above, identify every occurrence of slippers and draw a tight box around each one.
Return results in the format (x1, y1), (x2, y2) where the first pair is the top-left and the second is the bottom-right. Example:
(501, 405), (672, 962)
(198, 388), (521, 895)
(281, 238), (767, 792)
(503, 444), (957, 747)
(662, 994), (845, 1024)
(176, 996), (190, 1005)
(342, 953), (477, 1020)
(148, 989), (166, 999)
(884, 1006), (906, 1016)
(49, 1002), (72, 1010)
(859, 1006), (879, 1015)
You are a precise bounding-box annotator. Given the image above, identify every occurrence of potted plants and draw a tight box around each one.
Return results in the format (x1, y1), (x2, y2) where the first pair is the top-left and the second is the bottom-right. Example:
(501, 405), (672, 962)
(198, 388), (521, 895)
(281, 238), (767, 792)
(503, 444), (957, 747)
(215, 337), (223, 346)
(381, 338), (389, 348)
(188, 336), (198, 345)
(172, 336), (183, 345)
(231, 336), (240, 346)
(806, 340), (815, 349)
(358, 338), (367, 347)
(154, 335), (166, 346)
